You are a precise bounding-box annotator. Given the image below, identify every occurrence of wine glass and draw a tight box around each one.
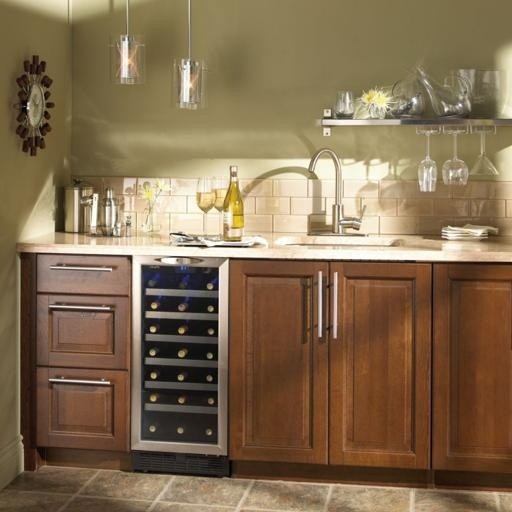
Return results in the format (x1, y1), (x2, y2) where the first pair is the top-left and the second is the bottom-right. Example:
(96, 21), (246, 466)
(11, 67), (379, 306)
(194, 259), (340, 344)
(416, 130), (501, 193)
(197, 174), (227, 239)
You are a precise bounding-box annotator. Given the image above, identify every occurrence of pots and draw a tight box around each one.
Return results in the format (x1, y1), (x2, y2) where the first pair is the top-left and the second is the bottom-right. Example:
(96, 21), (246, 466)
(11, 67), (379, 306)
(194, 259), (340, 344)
(62, 177), (97, 235)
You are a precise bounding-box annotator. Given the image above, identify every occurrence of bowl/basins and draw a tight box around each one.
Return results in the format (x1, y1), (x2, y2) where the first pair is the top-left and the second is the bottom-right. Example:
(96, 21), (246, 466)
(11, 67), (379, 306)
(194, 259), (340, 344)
(447, 67), (506, 118)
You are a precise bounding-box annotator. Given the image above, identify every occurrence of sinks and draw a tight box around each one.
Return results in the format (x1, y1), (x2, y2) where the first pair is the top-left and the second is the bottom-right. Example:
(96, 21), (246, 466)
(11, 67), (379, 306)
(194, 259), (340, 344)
(274, 235), (443, 252)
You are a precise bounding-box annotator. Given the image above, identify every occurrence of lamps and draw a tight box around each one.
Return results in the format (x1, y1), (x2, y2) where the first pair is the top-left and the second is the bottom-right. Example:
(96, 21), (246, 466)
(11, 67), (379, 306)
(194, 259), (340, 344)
(105, 1), (148, 88)
(170, 0), (209, 113)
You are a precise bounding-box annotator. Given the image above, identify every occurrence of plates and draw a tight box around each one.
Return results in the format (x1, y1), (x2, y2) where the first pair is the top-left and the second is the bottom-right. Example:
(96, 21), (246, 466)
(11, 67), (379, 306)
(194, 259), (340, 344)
(440, 227), (489, 242)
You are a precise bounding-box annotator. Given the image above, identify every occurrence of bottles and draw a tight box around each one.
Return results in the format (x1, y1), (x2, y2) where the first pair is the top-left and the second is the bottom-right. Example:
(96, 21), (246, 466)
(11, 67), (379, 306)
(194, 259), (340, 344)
(100, 187), (119, 236)
(145, 271), (217, 440)
(126, 215), (131, 237)
(221, 164), (248, 242)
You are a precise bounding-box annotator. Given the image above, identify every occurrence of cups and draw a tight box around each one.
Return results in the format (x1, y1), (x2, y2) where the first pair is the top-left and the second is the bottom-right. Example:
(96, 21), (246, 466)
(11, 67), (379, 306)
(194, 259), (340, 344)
(334, 88), (354, 117)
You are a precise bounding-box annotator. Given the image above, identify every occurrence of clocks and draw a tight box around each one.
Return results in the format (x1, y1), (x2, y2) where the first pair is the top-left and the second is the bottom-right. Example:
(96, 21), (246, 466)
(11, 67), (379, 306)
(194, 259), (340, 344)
(13, 52), (56, 158)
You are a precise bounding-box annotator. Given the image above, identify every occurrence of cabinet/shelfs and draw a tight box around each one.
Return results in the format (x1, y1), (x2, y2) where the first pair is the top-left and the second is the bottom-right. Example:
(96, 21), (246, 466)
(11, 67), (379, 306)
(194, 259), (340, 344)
(18, 253), (132, 475)
(228, 255), (433, 488)
(132, 253), (231, 478)
(430, 261), (512, 494)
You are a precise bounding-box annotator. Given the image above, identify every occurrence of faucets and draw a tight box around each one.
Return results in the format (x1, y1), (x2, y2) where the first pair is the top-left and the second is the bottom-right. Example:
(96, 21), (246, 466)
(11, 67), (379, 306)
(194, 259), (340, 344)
(307, 148), (367, 235)
